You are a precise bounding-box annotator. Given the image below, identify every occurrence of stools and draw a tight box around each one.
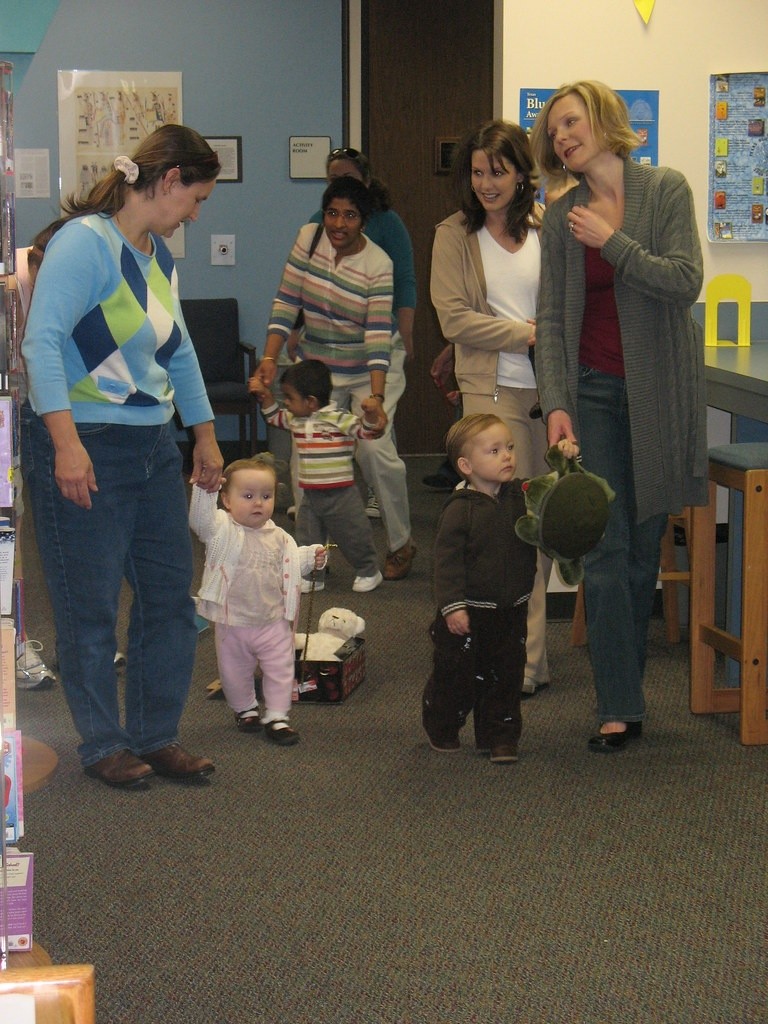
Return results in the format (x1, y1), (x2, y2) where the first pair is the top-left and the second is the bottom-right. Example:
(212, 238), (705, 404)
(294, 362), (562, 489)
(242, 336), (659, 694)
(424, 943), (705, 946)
(570, 504), (692, 648)
(689, 442), (767, 746)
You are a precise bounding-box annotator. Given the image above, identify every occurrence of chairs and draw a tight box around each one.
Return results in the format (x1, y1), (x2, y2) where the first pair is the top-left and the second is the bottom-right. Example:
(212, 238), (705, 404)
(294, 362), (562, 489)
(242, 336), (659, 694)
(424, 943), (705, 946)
(177, 298), (258, 465)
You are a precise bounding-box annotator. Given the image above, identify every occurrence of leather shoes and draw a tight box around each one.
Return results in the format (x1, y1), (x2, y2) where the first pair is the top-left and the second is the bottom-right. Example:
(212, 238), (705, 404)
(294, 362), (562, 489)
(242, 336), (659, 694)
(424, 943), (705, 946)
(136, 743), (216, 779)
(84, 750), (154, 788)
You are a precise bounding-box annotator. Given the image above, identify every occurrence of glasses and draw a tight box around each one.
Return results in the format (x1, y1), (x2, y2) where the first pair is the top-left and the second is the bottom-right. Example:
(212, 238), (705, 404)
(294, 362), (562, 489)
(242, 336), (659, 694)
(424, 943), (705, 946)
(328, 148), (360, 158)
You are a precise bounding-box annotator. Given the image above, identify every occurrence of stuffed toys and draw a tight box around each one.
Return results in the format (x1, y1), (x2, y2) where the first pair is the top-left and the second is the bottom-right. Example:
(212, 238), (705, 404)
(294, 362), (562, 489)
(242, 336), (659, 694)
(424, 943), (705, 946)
(513, 444), (614, 584)
(295, 606), (366, 661)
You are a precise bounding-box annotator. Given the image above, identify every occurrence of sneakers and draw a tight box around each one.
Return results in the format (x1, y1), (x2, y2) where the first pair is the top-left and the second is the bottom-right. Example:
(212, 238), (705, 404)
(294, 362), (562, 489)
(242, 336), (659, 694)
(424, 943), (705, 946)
(15, 634), (55, 692)
(113, 652), (126, 667)
(366, 493), (383, 518)
(300, 574), (323, 593)
(353, 573), (383, 592)
(288, 506), (298, 519)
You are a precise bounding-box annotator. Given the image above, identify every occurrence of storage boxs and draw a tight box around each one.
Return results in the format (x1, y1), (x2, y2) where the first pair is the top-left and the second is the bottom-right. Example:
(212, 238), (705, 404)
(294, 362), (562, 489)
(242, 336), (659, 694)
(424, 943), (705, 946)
(291, 638), (366, 703)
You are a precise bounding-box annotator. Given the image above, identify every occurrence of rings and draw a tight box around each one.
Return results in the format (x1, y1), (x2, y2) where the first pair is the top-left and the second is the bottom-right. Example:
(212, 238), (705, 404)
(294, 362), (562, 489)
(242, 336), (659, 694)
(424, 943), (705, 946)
(571, 224), (576, 230)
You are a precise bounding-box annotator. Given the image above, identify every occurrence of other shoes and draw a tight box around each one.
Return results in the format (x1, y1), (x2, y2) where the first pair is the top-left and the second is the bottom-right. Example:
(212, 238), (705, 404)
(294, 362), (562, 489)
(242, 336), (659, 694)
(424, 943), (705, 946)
(423, 472), (455, 491)
(520, 675), (548, 699)
(236, 707), (261, 731)
(385, 534), (417, 580)
(423, 712), (463, 752)
(586, 716), (643, 754)
(491, 741), (517, 763)
(265, 720), (300, 745)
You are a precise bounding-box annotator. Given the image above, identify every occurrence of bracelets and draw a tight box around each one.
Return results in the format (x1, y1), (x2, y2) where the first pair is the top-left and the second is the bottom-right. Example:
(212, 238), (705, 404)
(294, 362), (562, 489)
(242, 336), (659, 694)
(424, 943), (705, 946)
(263, 357), (275, 360)
(372, 394), (384, 401)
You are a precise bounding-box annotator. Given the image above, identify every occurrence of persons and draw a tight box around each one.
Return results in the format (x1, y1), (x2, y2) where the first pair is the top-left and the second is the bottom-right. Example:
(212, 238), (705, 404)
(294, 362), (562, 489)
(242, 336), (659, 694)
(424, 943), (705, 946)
(249, 360), (385, 592)
(421, 413), (580, 763)
(259, 176), (418, 582)
(18, 123), (224, 787)
(13, 224), (127, 691)
(285, 147), (417, 519)
(426, 345), (464, 489)
(531, 82), (709, 752)
(189, 453), (328, 746)
(429, 119), (556, 698)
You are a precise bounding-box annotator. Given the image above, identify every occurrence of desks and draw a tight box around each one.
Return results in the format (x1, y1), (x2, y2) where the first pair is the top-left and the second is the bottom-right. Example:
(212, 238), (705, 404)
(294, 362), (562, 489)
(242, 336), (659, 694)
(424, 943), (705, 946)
(702, 343), (768, 687)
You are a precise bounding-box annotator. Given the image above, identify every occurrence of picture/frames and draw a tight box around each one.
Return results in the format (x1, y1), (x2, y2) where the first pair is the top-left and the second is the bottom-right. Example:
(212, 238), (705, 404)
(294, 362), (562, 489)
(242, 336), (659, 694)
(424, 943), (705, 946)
(201, 136), (242, 184)
(288, 135), (331, 180)
